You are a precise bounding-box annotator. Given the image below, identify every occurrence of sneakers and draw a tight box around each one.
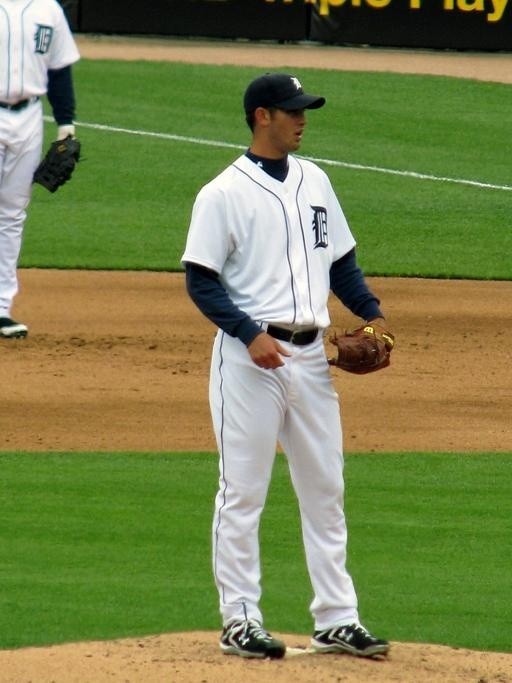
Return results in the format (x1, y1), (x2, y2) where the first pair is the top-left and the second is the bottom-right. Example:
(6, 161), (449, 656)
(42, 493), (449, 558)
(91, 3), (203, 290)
(0, 317), (27, 337)
(311, 622), (389, 656)
(218, 621), (285, 658)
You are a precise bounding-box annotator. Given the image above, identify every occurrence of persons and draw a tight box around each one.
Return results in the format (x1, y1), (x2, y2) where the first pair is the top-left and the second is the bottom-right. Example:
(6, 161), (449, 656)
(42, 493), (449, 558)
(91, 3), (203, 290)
(180, 76), (391, 660)
(0, 0), (82, 339)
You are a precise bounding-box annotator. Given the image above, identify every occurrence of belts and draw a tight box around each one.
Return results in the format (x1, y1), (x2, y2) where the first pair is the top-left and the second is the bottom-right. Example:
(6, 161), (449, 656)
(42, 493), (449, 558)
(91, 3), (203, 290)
(0, 99), (30, 111)
(268, 324), (318, 346)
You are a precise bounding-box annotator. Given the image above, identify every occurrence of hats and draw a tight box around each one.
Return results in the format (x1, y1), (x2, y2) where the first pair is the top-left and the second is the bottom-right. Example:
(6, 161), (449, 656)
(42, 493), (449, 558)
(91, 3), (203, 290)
(244, 73), (325, 112)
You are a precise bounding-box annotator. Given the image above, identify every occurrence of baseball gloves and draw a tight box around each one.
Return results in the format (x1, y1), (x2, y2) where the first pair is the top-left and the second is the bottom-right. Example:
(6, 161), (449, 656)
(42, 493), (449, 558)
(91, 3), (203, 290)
(32, 134), (80, 193)
(328, 323), (394, 374)
